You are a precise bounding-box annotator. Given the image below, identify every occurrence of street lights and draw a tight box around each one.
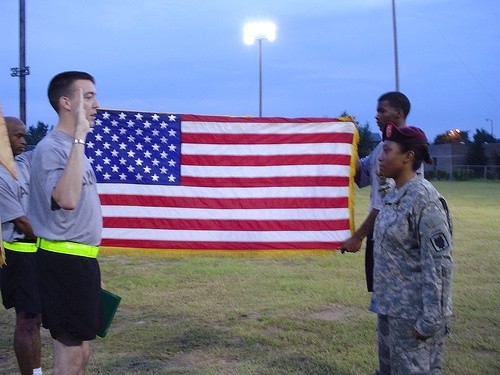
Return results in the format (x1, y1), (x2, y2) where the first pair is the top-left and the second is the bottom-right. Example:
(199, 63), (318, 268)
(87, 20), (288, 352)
(243, 21), (275, 117)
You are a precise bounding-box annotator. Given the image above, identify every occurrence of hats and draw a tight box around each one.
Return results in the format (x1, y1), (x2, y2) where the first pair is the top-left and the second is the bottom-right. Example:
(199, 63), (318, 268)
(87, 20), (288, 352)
(382, 122), (428, 144)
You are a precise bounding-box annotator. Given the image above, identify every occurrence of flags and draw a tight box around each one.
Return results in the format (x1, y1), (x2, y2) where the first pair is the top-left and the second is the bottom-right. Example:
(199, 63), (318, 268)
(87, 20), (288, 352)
(81, 107), (361, 258)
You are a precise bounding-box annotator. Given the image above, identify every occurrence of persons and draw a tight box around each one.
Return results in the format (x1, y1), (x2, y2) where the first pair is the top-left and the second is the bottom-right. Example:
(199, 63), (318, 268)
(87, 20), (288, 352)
(337, 90), (427, 292)
(368, 122), (454, 374)
(0, 115), (46, 375)
(28, 71), (109, 375)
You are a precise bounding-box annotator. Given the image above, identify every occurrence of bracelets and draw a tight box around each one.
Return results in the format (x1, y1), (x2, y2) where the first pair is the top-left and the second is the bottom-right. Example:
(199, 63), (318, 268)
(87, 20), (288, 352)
(71, 138), (86, 146)
